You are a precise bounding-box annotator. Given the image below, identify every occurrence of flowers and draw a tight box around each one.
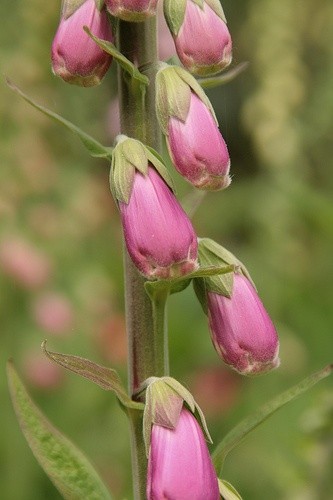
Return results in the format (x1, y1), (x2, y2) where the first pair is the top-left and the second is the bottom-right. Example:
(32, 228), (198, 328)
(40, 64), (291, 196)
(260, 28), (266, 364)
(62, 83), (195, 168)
(49, 1), (282, 499)
